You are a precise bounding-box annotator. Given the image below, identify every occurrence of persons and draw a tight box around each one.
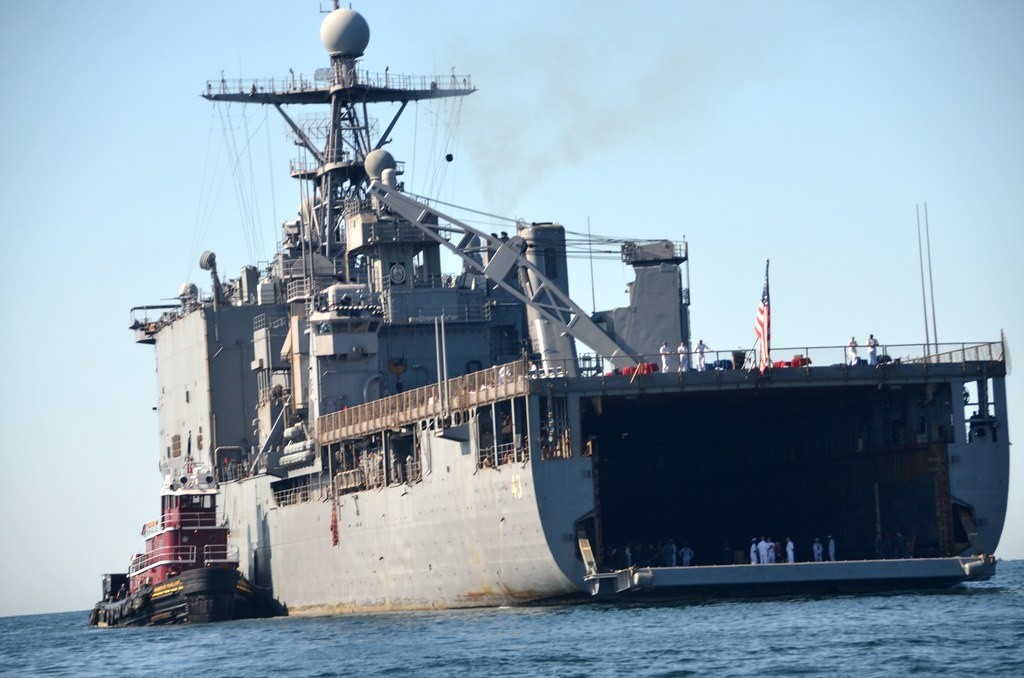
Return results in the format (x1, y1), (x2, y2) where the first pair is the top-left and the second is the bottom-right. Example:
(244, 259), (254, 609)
(866, 334), (879, 366)
(116, 584), (126, 599)
(660, 341), (675, 373)
(849, 337), (858, 366)
(503, 449), (513, 464)
(677, 342), (688, 372)
(695, 340), (709, 372)
(490, 363), (511, 388)
(608, 535), (836, 565)
(483, 457), (491, 468)
(528, 360), (537, 374)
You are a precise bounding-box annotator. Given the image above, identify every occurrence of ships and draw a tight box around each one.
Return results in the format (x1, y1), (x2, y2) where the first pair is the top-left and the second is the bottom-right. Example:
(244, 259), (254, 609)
(129, 1), (1011, 618)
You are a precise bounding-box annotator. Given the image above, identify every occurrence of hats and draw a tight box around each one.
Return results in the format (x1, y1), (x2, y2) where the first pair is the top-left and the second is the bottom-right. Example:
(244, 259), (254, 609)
(224, 458), (229, 463)
(169, 567), (172, 570)
(122, 584), (125, 587)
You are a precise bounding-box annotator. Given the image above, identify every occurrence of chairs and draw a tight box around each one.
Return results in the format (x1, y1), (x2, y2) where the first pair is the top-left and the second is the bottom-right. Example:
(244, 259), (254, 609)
(526, 366), (569, 379)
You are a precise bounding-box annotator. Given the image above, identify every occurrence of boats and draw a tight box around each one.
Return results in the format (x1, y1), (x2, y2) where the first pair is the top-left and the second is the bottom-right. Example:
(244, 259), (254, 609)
(86, 429), (290, 628)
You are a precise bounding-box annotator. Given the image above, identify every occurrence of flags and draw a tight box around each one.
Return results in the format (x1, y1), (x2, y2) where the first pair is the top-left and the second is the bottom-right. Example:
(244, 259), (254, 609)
(755, 263), (771, 375)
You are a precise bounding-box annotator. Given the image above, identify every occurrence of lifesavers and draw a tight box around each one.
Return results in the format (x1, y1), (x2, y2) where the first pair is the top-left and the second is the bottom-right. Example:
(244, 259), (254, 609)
(168, 564), (181, 575)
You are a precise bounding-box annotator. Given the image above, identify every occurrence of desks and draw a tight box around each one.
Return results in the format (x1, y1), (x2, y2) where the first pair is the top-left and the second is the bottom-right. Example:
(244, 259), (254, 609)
(831, 354), (892, 366)
(688, 359), (733, 371)
(773, 354), (812, 367)
(605, 362), (657, 377)
